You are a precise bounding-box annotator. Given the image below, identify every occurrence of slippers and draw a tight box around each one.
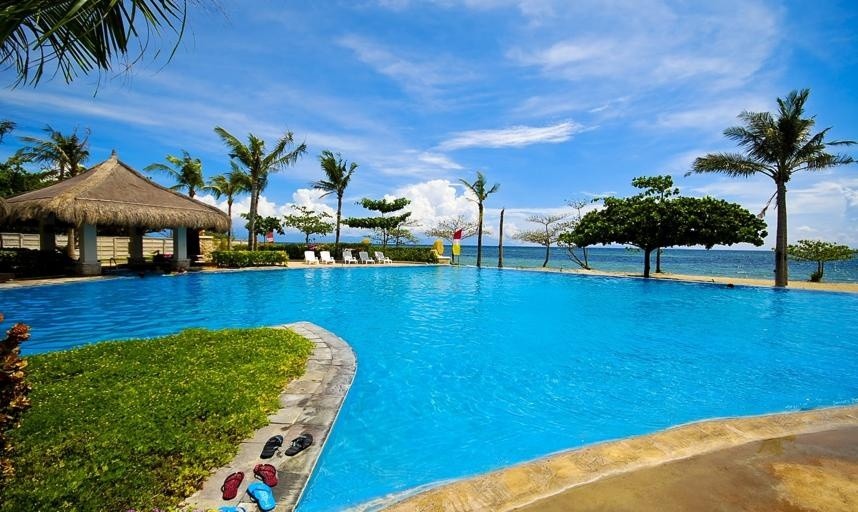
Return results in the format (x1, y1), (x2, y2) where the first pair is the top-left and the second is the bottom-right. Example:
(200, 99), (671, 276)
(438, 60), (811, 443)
(218, 507), (246, 512)
(284, 434), (313, 456)
(260, 435), (283, 458)
(255, 464), (277, 486)
(221, 472), (244, 500)
(248, 483), (276, 511)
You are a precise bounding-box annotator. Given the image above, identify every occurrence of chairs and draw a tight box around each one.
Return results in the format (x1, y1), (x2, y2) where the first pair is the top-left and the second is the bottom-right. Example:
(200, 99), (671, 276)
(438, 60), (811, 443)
(303, 249), (393, 264)
